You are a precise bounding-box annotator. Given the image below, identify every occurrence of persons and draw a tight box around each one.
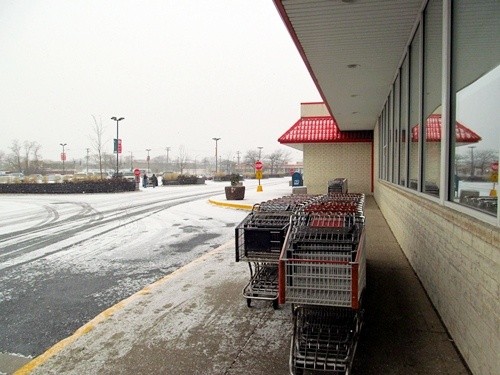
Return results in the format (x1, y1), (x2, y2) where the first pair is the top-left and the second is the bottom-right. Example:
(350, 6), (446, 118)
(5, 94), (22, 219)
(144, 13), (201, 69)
(143, 175), (147, 187)
(151, 174), (157, 188)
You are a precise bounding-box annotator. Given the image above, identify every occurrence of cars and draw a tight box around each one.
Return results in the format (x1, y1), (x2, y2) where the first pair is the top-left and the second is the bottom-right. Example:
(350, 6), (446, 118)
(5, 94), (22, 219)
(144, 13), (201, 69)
(82, 168), (133, 176)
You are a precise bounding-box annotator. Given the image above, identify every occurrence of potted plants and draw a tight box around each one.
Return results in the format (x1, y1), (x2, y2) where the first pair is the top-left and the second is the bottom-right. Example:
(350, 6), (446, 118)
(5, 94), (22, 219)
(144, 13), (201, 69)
(225, 173), (246, 200)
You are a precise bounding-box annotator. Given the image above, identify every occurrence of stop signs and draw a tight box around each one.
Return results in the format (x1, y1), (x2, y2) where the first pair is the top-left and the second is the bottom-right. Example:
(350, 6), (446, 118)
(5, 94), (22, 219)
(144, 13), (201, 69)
(254, 162), (263, 170)
(134, 169), (141, 176)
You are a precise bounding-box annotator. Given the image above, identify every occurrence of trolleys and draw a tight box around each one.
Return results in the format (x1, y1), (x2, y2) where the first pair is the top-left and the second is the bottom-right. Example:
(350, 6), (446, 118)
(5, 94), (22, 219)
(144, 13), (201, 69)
(462, 195), (497, 214)
(234, 192), (368, 375)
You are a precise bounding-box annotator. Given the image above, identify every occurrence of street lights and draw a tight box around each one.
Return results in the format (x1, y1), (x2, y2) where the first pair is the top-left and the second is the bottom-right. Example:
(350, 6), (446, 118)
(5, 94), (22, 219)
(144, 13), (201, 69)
(212, 137), (221, 173)
(60, 143), (68, 175)
(111, 116), (125, 180)
(257, 147), (264, 161)
